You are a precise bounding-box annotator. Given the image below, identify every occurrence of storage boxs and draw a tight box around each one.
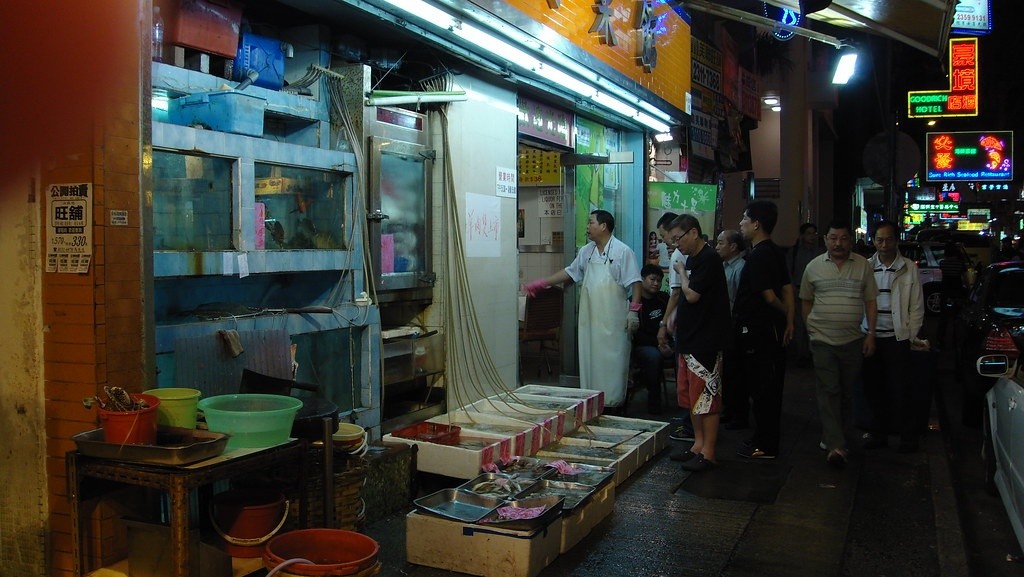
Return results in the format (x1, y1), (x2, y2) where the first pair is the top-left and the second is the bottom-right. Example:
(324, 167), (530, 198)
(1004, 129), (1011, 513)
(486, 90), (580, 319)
(151, 0), (244, 57)
(383, 384), (671, 577)
(167, 89), (267, 136)
(121, 516), (232, 577)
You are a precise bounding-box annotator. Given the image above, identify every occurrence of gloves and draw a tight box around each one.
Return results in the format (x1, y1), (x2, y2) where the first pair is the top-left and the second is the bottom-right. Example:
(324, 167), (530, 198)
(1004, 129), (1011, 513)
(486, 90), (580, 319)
(624, 303), (642, 341)
(523, 279), (547, 299)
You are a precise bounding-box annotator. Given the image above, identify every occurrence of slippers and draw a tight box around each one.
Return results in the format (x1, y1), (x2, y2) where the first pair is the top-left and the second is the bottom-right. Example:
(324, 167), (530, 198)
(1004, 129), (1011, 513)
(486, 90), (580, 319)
(670, 448), (696, 461)
(680, 453), (720, 471)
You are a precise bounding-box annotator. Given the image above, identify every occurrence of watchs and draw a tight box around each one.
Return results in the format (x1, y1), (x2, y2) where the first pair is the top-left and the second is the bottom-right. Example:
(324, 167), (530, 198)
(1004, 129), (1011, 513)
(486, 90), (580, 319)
(659, 321), (667, 327)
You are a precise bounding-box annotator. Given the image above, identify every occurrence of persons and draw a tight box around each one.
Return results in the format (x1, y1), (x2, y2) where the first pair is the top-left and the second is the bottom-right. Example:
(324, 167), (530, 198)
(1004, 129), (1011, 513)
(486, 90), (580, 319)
(629, 264), (679, 415)
(859, 221), (924, 452)
(799, 222), (881, 461)
(524, 210), (644, 415)
(657, 200), (794, 471)
(793, 224), (818, 367)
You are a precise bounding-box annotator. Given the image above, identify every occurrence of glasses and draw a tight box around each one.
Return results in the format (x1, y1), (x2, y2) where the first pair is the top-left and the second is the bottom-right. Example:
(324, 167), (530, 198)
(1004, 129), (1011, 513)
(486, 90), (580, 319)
(671, 227), (692, 246)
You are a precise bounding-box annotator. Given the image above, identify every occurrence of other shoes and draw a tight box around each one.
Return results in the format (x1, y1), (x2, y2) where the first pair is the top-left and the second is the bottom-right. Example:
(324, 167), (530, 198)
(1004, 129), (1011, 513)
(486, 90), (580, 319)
(736, 445), (777, 458)
(669, 424), (695, 441)
(720, 422), (751, 432)
(896, 440), (918, 454)
(743, 439), (755, 447)
(862, 435), (887, 451)
(647, 408), (662, 415)
(820, 441), (828, 451)
(827, 448), (847, 467)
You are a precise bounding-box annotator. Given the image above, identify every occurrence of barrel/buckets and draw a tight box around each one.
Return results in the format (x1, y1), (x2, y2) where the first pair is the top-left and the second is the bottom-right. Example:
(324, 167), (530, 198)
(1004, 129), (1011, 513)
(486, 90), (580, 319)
(311, 423), (368, 536)
(262, 528), (382, 577)
(97, 394), (161, 445)
(144, 388), (201, 430)
(208, 489), (291, 558)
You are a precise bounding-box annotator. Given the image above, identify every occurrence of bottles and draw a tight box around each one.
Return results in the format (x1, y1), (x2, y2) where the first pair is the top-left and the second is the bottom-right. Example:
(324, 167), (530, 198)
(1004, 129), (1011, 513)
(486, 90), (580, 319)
(152, 6), (165, 64)
(518, 212), (524, 237)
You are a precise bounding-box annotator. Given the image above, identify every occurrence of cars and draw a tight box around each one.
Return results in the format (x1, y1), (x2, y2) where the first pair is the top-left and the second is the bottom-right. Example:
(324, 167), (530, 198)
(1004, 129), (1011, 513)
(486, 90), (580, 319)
(900, 228), (1024, 429)
(975, 353), (1024, 562)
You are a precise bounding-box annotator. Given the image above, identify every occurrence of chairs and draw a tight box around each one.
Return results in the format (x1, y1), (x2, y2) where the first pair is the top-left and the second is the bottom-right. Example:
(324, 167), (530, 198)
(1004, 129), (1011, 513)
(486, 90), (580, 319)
(518, 283), (563, 381)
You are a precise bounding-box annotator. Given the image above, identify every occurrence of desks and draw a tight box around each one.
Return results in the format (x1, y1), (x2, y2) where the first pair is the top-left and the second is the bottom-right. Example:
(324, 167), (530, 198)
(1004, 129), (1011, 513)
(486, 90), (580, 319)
(65, 436), (307, 577)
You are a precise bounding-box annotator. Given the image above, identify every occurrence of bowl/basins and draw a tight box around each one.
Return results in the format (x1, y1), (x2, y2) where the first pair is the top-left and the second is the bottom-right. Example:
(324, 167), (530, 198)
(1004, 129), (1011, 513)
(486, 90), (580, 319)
(197, 393), (303, 448)
